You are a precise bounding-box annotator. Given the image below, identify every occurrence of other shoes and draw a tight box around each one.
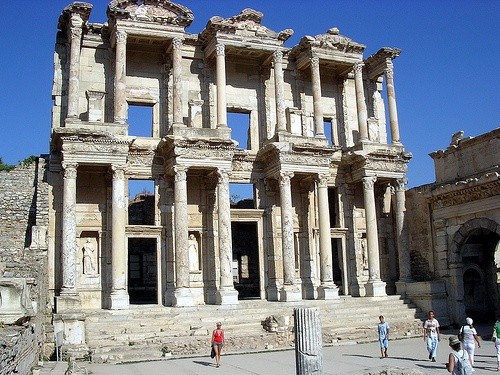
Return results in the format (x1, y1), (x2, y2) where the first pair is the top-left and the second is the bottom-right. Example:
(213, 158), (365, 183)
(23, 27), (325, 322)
(429, 352), (432, 359)
(432, 357), (436, 362)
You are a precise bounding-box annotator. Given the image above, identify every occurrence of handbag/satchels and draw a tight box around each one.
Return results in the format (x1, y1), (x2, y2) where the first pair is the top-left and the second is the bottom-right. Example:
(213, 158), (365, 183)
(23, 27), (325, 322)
(210, 344), (216, 359)
(458, 325), (464, 341)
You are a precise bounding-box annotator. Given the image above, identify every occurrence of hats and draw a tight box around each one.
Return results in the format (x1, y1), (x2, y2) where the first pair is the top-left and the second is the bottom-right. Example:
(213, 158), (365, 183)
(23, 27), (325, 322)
(448, 336), (461, 346)
(466, 317), (473, 325)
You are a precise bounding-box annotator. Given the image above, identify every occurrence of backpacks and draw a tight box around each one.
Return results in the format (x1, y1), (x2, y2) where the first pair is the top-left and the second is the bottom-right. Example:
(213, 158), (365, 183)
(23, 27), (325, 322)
(452, 349), (472, 375)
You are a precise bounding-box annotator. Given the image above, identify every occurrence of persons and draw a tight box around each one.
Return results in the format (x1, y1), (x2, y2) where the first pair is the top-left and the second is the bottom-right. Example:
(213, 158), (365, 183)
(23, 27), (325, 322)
(493, 315), (500, 371)
(377, 315), (389, 359)
(458, 317), (480, 372)
(211, 322), (225, 368)
(423, 310), (440, 362)
(445, 335), (470, 375)
